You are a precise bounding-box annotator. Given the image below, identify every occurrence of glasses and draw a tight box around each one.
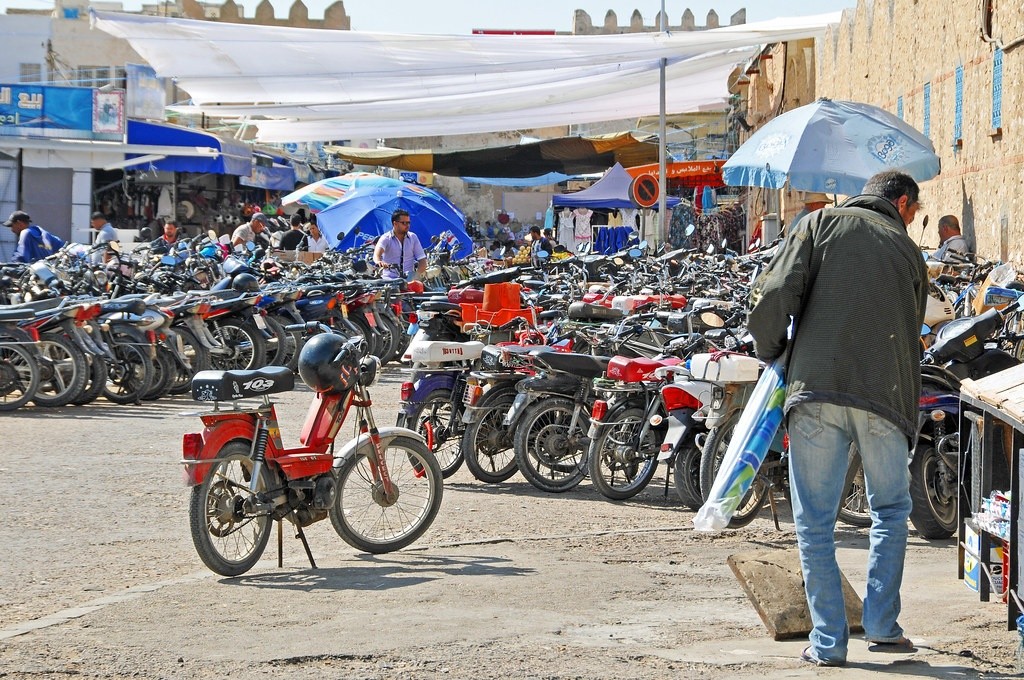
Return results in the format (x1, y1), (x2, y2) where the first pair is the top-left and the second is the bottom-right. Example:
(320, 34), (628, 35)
(396, 221), (410, 225)
(259, 222), (265, 230)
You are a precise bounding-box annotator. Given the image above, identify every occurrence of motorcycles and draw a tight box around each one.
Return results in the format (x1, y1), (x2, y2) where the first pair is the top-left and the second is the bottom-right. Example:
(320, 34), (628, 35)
(181, 307), (443, 576)
(396, 215), (1024, 539)
(0, 241), (407, 411)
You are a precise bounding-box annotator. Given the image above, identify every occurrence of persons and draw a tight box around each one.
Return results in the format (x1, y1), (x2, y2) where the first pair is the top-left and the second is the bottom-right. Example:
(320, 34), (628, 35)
(373, 210), (428, 282)
(618, 207), (656, 249)
(90, 212), (116, 265)
(933, 215), (967, 262)
(558, 207), (593, 250)
(544, 228), (560, 248)
(789, 193), (833, 233)
(746, 171), (929, 666)
(279, 213), (308, 252)
(161, 220), (178, 243)
(485, 222), (499, 238)
(490, 240), (519, 258)
(3, 211), (64, 263)
(307, 221), (328, 252)
(231, 213), (267, 245)
(530, 226), (553, 255)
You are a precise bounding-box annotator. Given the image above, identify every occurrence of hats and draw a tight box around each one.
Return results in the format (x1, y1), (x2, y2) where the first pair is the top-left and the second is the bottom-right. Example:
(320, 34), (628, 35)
(2, 211), (32, 227)
(799, 192), (834, 204)
(252, 212), (271, 227)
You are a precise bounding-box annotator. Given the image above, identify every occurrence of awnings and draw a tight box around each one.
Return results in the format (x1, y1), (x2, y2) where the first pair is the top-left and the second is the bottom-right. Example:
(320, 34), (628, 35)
(125, 120), (252, 178)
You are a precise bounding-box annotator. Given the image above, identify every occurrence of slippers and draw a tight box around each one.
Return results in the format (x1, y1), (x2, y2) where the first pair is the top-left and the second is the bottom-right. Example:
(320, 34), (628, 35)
(800, 644), (846, 667)
(868, 638), (918, 653)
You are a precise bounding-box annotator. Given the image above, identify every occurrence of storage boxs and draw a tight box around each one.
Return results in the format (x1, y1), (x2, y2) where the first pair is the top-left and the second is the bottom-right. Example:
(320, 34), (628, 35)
(458, 303), (541, 328)
(964, 518), (1003, 593)
(273, 249), (323, 265)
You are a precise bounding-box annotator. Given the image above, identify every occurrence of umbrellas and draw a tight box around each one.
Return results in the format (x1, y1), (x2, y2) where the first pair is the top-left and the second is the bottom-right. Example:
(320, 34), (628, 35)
(723, 97), (942, 207)
(315, 184), (474, 261)
(280, 171), (408, 214)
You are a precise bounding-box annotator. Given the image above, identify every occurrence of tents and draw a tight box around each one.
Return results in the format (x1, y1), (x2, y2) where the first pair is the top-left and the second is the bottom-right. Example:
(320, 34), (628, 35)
(552, 162), (681, 209)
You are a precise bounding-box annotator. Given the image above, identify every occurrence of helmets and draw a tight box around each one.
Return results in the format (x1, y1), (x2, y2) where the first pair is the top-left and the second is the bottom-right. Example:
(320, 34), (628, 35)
(297, 333), (360, 399)
(231, 273), (259, 294)
